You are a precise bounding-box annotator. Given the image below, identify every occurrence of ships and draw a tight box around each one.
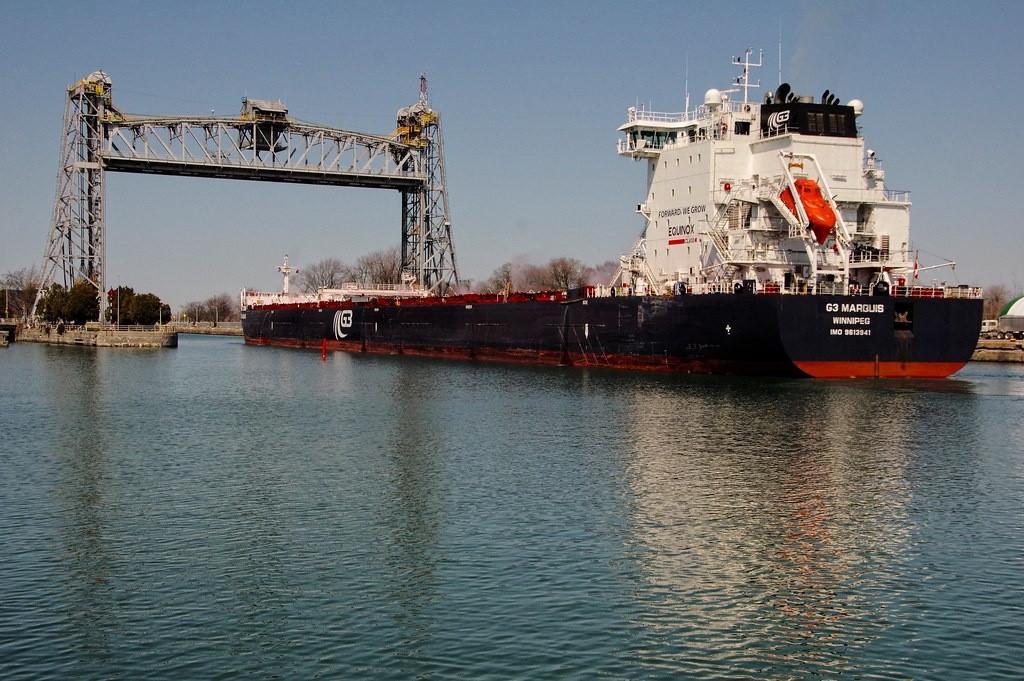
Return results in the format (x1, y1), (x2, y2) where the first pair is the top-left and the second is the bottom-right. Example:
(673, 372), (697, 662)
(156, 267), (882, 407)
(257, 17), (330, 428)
(240, 15), (989, 379)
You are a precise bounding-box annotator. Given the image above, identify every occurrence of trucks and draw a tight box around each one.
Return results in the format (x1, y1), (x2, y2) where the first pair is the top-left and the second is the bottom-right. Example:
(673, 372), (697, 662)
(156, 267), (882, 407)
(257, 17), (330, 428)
(979, 315), (1024, 339)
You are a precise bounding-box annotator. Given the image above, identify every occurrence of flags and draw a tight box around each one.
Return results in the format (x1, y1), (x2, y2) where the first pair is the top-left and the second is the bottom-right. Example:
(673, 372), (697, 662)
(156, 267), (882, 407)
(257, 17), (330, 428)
(913, 250), (919, 283)
